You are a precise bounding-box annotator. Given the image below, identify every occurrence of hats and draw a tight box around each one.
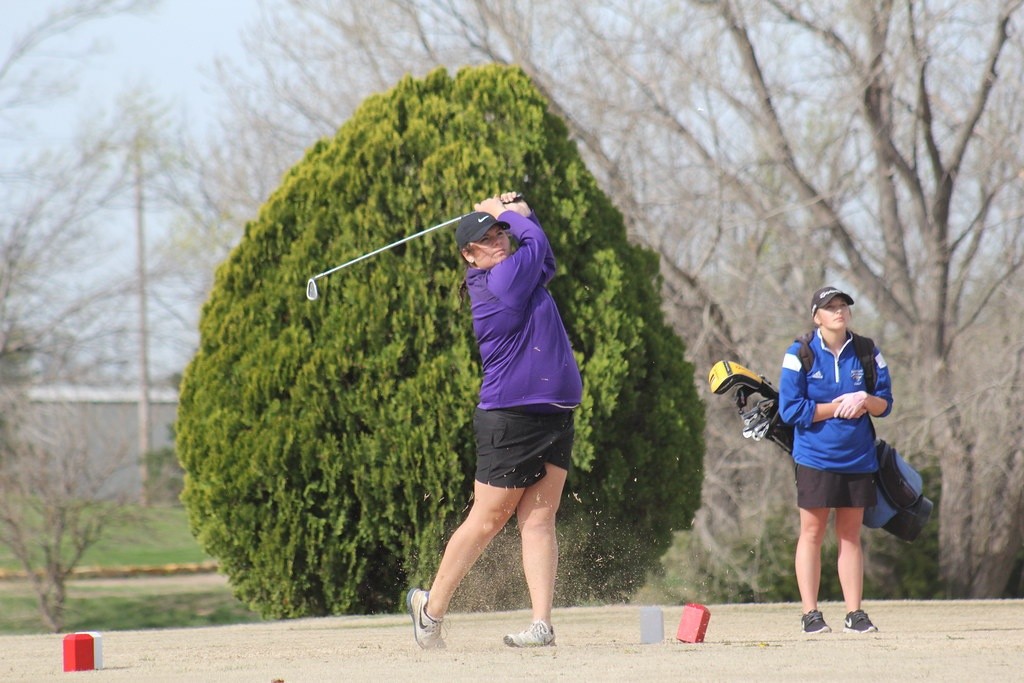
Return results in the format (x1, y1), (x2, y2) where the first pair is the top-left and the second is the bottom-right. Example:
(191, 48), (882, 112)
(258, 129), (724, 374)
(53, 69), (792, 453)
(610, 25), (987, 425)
(455, 212), (511, 252)
(811, 286), (854, 318)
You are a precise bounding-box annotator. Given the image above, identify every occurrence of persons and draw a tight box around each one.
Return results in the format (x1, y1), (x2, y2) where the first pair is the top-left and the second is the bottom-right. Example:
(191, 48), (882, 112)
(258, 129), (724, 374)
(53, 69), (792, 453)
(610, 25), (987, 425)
(778, 287), (894, 635)
(407, 190), (583, 649)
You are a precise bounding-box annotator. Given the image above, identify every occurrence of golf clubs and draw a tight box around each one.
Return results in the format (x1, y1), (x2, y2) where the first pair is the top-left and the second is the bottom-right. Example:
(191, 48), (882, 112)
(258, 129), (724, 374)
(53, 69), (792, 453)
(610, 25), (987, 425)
(740, 398), (777, 442)
(307, 191), (522, 299)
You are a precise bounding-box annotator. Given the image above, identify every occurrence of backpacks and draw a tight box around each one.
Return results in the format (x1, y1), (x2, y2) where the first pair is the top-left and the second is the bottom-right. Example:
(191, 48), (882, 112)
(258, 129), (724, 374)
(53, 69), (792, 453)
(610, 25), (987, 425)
(765, 330), (934, 544)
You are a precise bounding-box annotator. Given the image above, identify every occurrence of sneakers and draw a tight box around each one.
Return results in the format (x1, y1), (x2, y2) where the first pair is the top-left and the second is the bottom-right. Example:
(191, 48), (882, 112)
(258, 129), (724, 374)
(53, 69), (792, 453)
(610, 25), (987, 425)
(843, 609), (878, 634)
(503, 620), (557, 648)
(406, 587), (451, 650)
(801, 609), (832, 634)
